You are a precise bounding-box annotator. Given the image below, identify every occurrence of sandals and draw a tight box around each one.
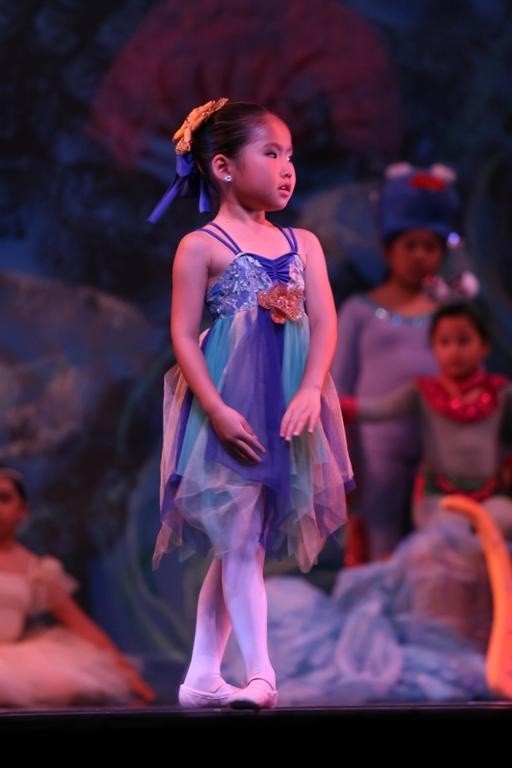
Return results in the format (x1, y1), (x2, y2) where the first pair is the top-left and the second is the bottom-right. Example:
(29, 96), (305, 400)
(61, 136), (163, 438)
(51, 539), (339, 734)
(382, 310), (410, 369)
(227, 675), (279, 709)
(177, 679), (244, 710)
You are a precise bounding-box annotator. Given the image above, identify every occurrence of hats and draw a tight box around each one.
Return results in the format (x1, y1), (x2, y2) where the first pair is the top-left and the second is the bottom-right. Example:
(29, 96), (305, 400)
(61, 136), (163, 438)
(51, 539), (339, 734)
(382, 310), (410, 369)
(373, 168), (458, 242)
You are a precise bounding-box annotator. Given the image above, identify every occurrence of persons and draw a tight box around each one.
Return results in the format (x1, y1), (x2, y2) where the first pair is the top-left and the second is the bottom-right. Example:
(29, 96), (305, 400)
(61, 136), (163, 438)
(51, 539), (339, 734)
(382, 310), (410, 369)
(222, 487), (508, 706)
(1, 464), (162, 714)
(328, 161), (467, 564)
(148, 93), (356, 714)
(335, 302), (512, 537)
